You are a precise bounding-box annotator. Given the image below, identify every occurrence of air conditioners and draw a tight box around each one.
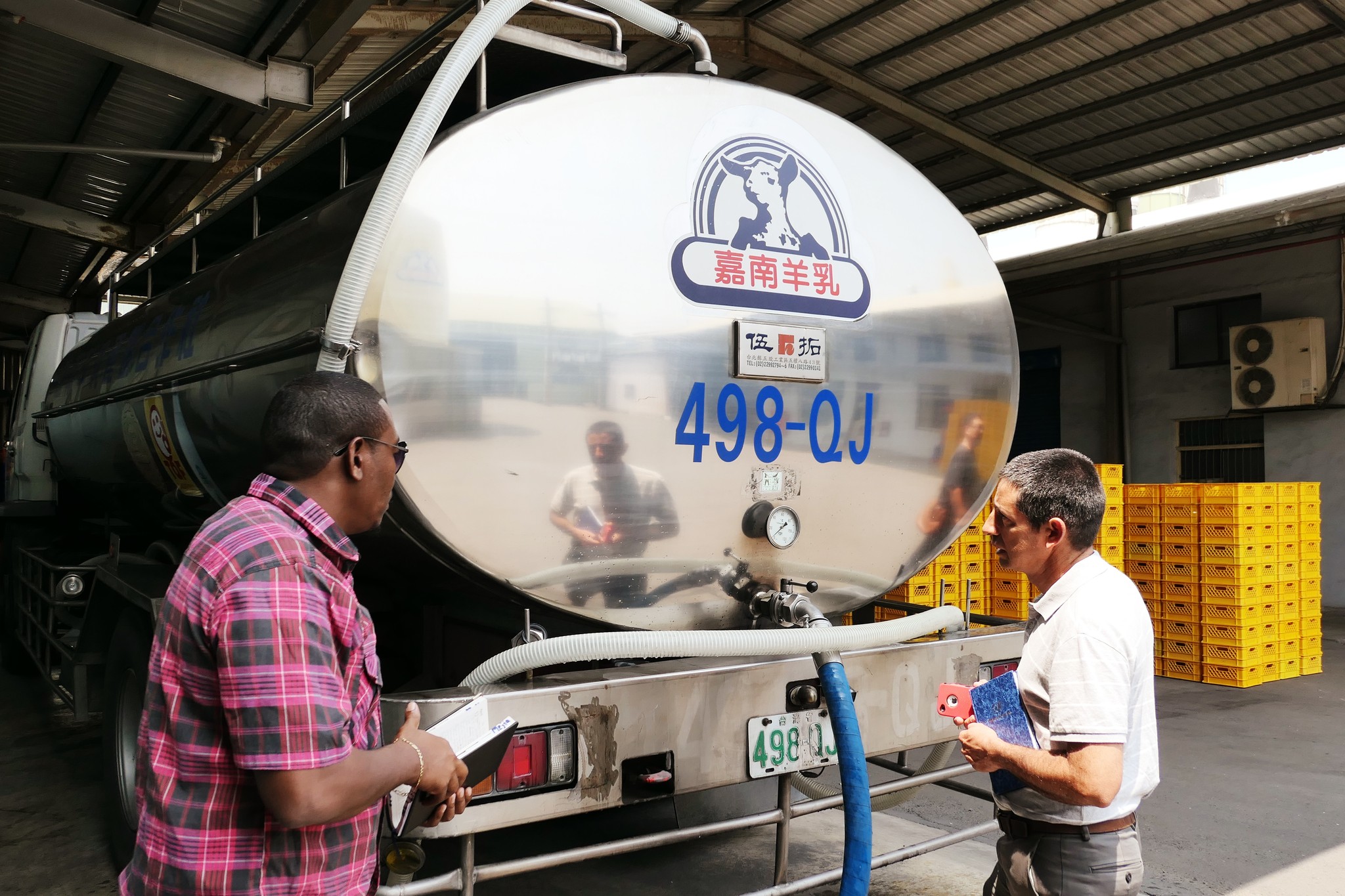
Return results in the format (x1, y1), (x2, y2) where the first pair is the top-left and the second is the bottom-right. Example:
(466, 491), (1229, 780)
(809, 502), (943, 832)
(1228, 316), (1327, 410)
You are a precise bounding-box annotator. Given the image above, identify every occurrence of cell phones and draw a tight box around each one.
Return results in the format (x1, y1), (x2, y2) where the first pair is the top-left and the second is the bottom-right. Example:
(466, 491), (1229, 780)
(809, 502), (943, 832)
(937, 682), (976, 720)
(599, 525), (613, 542)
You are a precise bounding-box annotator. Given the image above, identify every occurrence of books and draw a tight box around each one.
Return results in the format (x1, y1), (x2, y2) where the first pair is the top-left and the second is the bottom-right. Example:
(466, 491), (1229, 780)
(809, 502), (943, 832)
(969, 670), (1041, 797)
(384, 692), (518, 839)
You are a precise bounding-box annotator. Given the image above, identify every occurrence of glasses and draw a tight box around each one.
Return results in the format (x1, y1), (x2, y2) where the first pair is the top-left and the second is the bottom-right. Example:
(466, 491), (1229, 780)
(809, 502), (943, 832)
(335, 436), (410, 474)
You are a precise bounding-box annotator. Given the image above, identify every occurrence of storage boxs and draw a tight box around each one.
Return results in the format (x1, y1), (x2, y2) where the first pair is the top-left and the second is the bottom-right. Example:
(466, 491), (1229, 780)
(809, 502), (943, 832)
(842, 463), (1322, 688)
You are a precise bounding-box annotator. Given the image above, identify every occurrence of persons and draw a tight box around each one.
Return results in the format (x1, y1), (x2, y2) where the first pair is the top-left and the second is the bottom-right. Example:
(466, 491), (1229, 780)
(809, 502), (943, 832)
(930, 414), (984, 551)
(117, 372), (473, 896)
(953, 447), (1159, 896)
(547, 421), (681, 608)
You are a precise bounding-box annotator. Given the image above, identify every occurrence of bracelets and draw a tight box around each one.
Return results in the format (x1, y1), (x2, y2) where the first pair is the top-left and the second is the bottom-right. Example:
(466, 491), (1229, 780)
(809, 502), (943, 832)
(393, 737), (424, 797)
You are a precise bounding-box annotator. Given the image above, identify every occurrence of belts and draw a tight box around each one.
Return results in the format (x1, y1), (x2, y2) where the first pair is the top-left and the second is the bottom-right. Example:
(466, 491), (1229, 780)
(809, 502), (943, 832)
(995, 808), (1138, 835)
